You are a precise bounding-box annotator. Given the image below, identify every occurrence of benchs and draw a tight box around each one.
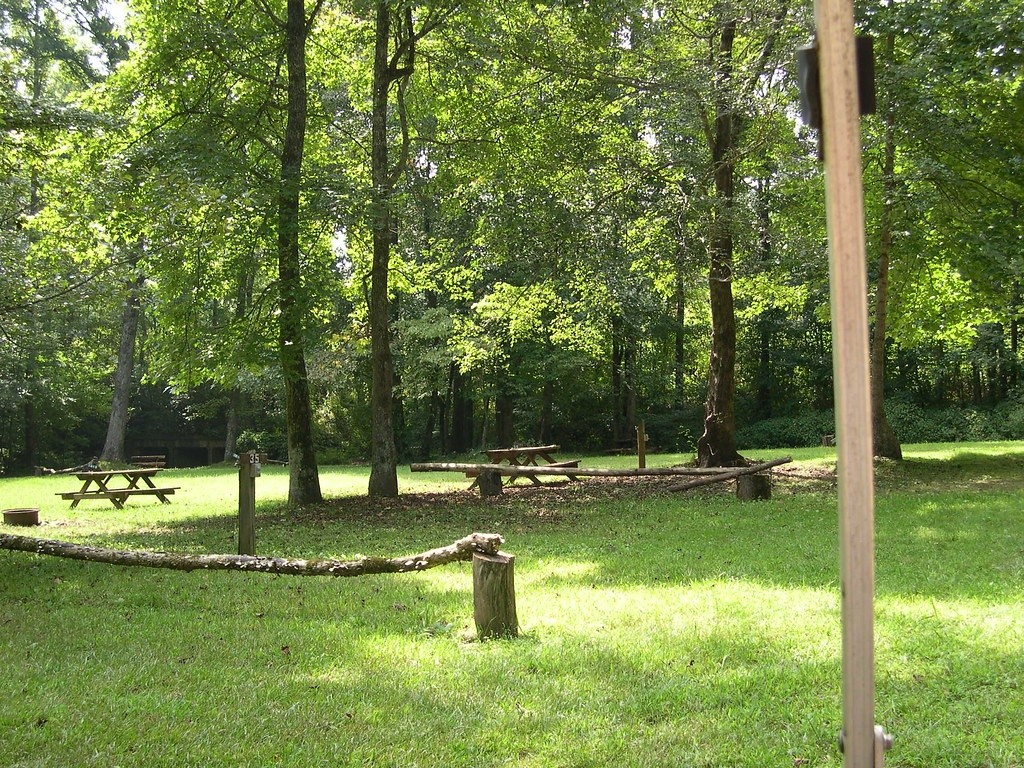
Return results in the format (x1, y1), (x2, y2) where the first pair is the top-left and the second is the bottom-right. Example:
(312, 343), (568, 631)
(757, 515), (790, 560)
(104, 486), (180, 491)
(55, 488), (126, 495)
(541, 459), (582, 465)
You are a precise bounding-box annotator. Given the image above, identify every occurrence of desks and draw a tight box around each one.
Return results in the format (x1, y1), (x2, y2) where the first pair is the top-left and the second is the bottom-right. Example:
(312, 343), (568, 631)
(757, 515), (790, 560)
(467, 445), (579, 488)
(69, 469), (171, 510)
